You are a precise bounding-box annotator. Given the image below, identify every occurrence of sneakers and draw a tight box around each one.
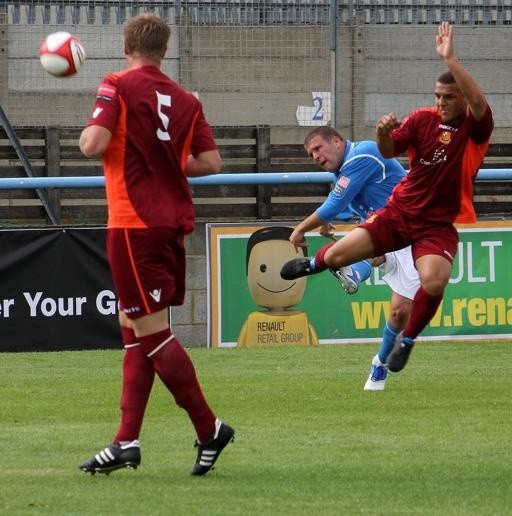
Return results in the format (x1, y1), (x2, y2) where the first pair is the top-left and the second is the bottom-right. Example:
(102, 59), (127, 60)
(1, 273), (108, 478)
(329, 265), (361, 294)
(280, 257), (315, 280)
(363, 354), (389, 391)
(79, 439), (140, 476)
(386, 330), (415, 372)
(191, 418), (235, 475)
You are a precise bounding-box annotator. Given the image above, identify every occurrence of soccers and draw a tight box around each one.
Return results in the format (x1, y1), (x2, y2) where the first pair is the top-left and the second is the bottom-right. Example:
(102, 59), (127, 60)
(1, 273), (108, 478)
(39, 31), (85, 77)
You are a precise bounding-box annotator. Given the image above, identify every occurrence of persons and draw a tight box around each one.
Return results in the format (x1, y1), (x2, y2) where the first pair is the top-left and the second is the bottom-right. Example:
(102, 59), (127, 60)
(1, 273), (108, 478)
(78, 13), (235, 477)
(279, 20), (496, 373)
(290, 125), (422, 392)
